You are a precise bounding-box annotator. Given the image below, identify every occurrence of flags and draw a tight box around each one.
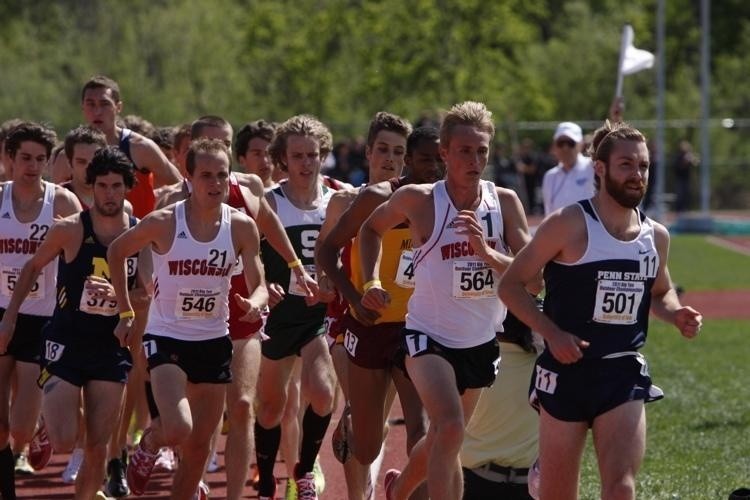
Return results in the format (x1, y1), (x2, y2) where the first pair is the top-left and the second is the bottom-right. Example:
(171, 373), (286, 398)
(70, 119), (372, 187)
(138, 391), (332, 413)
(615, 26), (655, 75)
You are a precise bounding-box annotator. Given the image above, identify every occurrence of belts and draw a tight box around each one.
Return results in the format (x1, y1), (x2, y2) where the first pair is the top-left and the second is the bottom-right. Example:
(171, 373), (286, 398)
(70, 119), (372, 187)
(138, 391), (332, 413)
(473, 468), (528, 484)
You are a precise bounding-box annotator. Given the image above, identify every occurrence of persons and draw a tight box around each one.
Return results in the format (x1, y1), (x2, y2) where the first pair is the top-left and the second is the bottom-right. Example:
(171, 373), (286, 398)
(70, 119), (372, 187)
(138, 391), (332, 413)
(2, 73), (707, 499)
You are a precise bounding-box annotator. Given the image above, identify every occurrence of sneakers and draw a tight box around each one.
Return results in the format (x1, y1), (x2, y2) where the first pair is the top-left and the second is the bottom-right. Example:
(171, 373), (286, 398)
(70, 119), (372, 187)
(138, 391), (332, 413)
(14, 413), (217, 497)
(251, 453), (324, 500)
(333, 407), (352, 463)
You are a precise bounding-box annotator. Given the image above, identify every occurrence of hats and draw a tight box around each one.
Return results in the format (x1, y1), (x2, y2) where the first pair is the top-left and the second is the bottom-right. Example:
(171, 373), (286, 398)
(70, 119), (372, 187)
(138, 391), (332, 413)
(553, 123), (582, 142)
